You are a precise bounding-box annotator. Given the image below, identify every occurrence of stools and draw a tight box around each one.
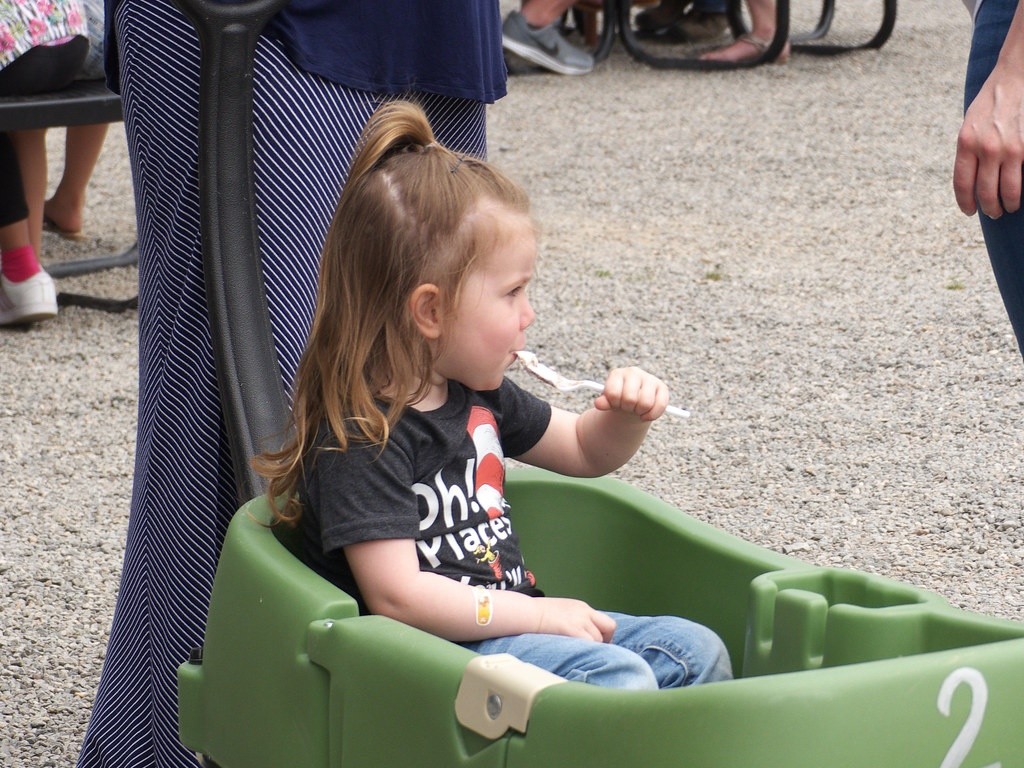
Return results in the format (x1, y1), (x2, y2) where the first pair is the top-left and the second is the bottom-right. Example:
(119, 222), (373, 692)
(0, 80), (141, 312)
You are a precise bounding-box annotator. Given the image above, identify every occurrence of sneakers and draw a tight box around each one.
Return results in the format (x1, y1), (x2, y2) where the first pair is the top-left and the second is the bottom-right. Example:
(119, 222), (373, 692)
(667, 11), (734, 42)
(502, 9), (595, 75)
(633, 1), (694, 32)
(2, 264), (58, 325)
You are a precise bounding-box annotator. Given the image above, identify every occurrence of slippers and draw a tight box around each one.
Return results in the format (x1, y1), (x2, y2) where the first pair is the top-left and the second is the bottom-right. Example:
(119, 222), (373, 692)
(700, 33), (792, 67)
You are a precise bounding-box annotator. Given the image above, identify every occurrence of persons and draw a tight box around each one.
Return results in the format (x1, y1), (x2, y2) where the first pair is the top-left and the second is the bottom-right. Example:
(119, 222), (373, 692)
(247, 102), (733, 692)
(72, 0), (507, 768)
(950, 0), (1024, 361)
(0, 0), (118, 330)
(500, 0), (796, 76)
